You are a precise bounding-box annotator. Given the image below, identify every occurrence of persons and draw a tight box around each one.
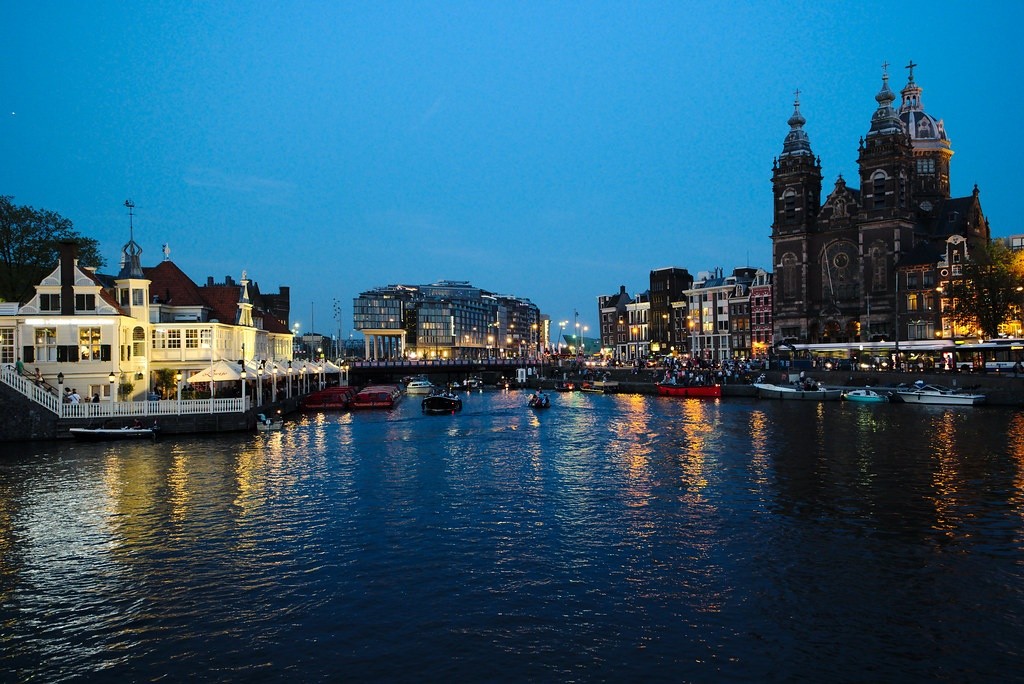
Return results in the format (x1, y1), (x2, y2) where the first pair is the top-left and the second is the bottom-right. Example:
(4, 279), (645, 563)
(551, 357), (622, 388)
(182, 384), (240, 397)
(531, 386), (549, 403)
(796, 370), (819, 391)
(62, 388), (81, 404)
(664, 357), (766, 385)
(82, 350), (100, 360)
(15, 357), (24, 377)
(34, 368), (43, 388)
(47, 387), (54, 394)
(1017, 358), (1022, 372)
(914, 355), (952, 368)
(91, 393), (100, 408)
(781, 373), (786, 387)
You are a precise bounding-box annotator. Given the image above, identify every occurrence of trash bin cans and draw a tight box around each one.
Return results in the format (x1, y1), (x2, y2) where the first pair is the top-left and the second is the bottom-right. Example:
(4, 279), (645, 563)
(148, 394), (158, 407)
(961, 364), (970, 374)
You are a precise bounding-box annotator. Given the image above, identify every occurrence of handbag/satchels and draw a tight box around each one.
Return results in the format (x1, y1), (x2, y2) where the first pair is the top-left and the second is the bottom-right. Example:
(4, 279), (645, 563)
(39, 377), (43, 381)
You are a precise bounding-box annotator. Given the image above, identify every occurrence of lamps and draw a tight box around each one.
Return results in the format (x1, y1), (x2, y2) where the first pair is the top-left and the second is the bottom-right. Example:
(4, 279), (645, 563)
(136, 373), (143, 380)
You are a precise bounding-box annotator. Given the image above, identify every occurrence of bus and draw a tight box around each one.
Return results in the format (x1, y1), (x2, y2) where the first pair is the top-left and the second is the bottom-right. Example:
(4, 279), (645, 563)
(954, 342), (1024, 377)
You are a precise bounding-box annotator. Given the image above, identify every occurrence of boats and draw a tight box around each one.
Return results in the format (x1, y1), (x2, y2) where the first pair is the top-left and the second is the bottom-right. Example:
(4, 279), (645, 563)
(752, 383), (844, 401)
(842, 389), (891, 405)
(496, 375), (529, 390)
(406, 377), (436, 395)
(896, 383), (987, 405)
(255, 413), (293, 433)
(306, 383), (401, 414)
(420, 386), (464, 414)
(528, 392), (551, 409)
(557, 382), (605, 394)
(655, 381), (723, 400)
(448, 375), (485, 390)
(68, 425), (158, 441)
